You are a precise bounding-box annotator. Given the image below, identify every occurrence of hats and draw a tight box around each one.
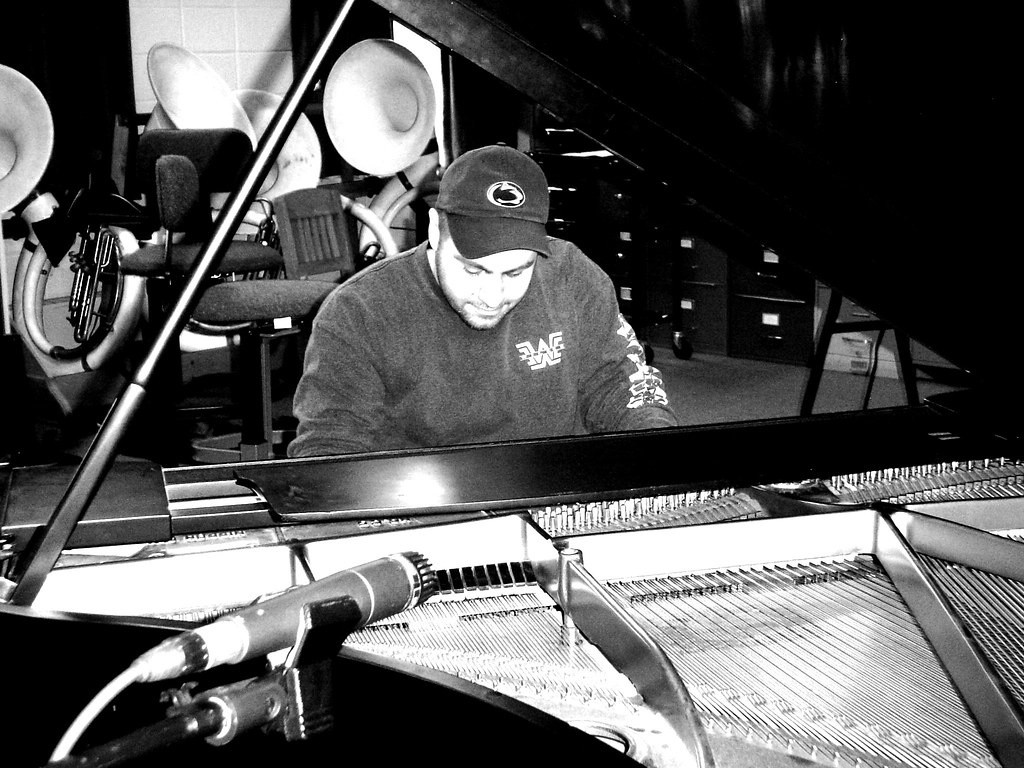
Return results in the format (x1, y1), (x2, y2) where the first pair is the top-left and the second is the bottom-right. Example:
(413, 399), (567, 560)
(435, 142), (553, 260)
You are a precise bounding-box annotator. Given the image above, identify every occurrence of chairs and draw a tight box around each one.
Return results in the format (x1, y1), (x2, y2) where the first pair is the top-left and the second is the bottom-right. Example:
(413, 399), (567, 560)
(159, 155), (346, 463)
(276, 190), (358, 362)
(120, 126), (284, 419)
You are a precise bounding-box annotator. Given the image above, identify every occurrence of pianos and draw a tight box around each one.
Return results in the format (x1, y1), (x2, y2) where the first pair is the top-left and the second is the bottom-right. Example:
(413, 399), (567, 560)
(1, 0), (1024, 768)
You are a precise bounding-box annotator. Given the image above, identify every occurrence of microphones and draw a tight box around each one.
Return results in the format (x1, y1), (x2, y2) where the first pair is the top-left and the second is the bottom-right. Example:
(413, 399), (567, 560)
(128, 552), (439, 681)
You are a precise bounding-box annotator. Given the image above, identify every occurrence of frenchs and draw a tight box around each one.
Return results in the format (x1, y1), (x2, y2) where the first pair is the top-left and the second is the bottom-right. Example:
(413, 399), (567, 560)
(324, 37), (466, 266)
(143, 42), (322, 287)
(0, 64), (146, 380)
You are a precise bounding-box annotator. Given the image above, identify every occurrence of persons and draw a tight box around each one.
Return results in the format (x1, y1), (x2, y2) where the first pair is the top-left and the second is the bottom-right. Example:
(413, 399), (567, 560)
(287, 142), (679, 460)
